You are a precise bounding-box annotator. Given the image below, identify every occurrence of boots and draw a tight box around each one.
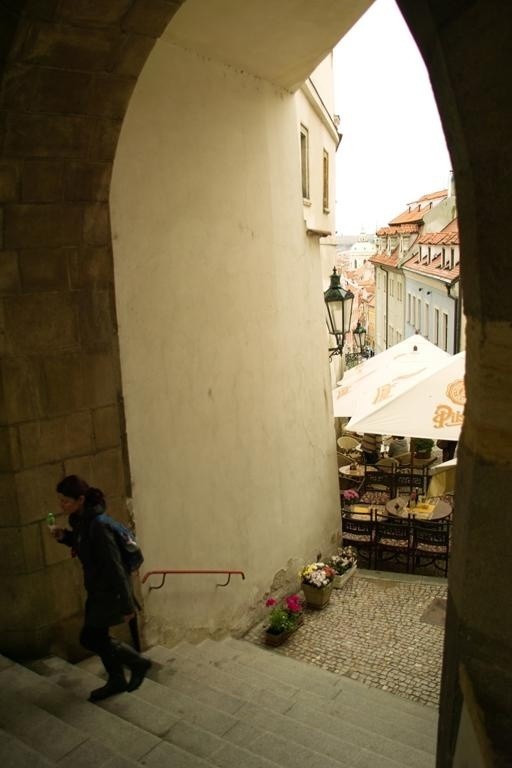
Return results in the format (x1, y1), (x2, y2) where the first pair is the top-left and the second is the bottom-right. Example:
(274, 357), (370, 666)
(108, 641), (152, 692)
(90, 654), (127, 701)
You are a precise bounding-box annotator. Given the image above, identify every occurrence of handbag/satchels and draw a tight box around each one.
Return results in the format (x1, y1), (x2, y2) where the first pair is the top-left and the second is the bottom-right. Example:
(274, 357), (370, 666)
(98, 514), (144, 573)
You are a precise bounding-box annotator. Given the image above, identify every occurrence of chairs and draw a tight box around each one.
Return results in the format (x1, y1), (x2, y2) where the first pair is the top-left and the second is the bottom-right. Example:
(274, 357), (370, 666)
(334, 417), (459, 578)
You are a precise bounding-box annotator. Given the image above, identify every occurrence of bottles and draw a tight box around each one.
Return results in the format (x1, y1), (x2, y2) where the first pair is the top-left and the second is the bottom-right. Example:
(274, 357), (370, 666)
(46, 511), (58, 536)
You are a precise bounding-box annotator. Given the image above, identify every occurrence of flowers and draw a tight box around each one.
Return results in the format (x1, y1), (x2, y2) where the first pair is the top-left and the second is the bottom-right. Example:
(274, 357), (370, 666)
(266, 594), (302, 632)
(298, 561), (335, 587)
(326, 545), (358, 575)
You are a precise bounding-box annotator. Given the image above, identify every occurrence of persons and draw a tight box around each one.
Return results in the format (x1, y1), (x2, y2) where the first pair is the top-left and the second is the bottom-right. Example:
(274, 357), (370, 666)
(48, 474), (151, 702)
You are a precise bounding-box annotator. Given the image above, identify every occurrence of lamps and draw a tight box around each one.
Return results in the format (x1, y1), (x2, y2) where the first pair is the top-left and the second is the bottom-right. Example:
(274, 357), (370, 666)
(320, 266), (354, 362)
(346, 319), (366, 355)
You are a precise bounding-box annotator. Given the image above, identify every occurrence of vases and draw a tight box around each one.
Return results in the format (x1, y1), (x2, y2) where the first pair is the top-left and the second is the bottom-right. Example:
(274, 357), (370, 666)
(332, 564), (358, 589)
(301, 581), (332, 609)
(265, 615), (304, 647)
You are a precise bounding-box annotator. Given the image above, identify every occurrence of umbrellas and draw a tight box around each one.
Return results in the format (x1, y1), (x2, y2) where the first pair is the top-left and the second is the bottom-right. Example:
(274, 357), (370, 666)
(332, 332), (466, 464)
